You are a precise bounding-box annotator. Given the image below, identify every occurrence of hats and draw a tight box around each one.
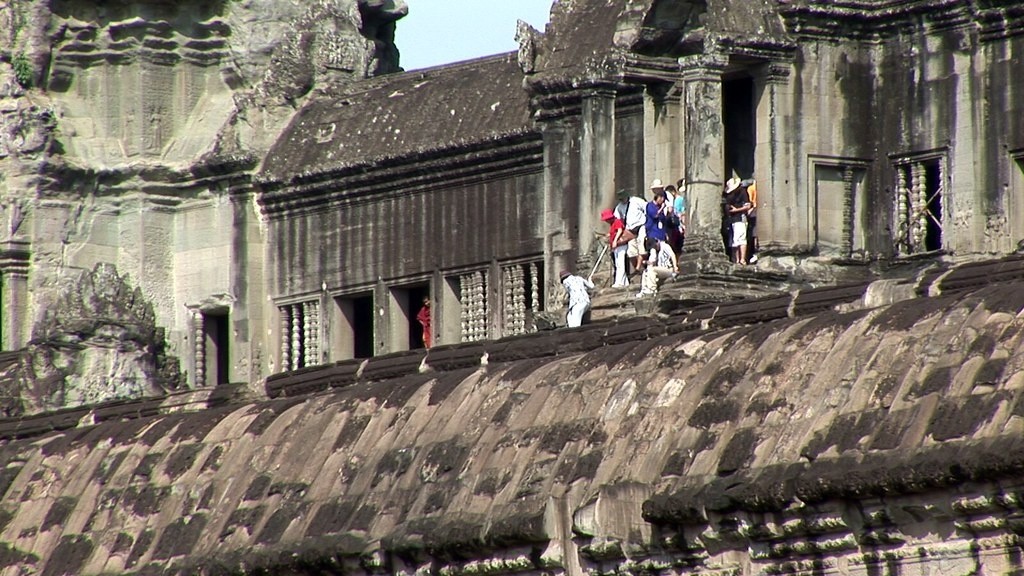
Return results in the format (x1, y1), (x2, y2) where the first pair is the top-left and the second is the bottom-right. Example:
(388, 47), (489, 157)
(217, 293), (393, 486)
(650, 179), (664, 188)
(617, 188), (629, 200)
(657, 190), (670, 202)
(600, 208), (614, 221)
(725, 177), (741, 194)
(560, 269), (571, 284)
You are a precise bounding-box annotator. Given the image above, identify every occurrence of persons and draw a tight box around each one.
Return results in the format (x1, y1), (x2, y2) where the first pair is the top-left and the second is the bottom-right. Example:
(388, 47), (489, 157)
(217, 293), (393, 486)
(648, 179), (675, 245)
(646, 190), (669, 273)
(613, 188), (649, 273)
(634, 236), (679, 299)
(559, 269), (596, 327)
(416, 295), (430, 348)
(600, 209), (630, 289)
(665, 185), (686, 237)
(721, 173), (758, 266)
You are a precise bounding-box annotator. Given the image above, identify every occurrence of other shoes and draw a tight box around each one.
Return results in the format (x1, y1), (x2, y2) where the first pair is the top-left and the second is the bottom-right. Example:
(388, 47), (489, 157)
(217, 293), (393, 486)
(612, 284), (628, 287)
(636, 293), (656, 298)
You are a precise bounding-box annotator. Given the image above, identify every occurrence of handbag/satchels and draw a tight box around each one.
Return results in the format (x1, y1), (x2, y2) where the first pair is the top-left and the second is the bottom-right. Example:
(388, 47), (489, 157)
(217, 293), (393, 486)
(673, 215), (679, 226)
(617, 230), (635, 245)
